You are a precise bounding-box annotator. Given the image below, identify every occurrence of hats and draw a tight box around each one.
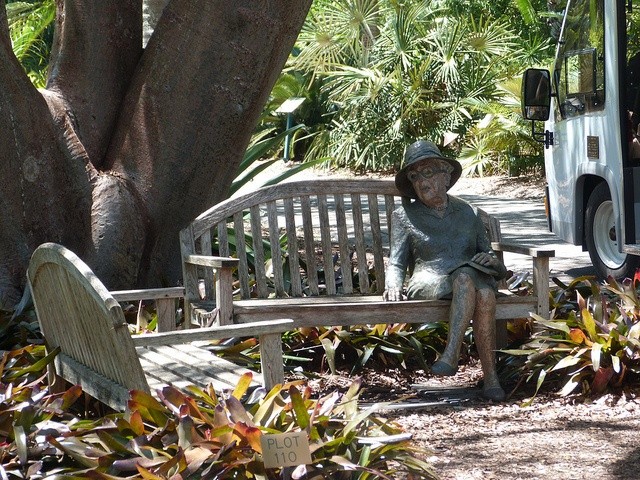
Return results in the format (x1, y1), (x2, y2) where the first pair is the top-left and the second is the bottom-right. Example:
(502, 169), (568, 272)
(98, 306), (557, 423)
(394, 139), (462, 198)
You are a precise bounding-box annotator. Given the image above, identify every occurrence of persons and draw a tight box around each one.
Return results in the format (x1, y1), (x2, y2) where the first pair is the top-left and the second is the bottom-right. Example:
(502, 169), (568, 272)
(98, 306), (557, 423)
(383, 140), (508, 403)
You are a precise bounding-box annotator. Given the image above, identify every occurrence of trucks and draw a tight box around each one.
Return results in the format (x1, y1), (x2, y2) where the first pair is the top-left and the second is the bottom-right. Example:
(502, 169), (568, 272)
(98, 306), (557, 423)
(521, 0), (640, 280)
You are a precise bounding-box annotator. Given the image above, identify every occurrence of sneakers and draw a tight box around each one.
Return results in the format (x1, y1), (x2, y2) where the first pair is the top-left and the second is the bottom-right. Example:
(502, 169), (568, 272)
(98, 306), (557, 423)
(485, 387), (505, 402)
(431, 361), (459, 375)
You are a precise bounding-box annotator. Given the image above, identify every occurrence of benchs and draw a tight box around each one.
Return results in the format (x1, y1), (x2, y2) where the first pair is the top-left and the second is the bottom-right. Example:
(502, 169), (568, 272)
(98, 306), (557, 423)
(180, 179), (555, 351)
(25, 241), (294, 421)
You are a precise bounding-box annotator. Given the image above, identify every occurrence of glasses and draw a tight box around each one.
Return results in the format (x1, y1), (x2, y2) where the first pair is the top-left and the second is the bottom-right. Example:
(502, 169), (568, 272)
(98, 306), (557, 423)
(407, 166), (447, 181)
